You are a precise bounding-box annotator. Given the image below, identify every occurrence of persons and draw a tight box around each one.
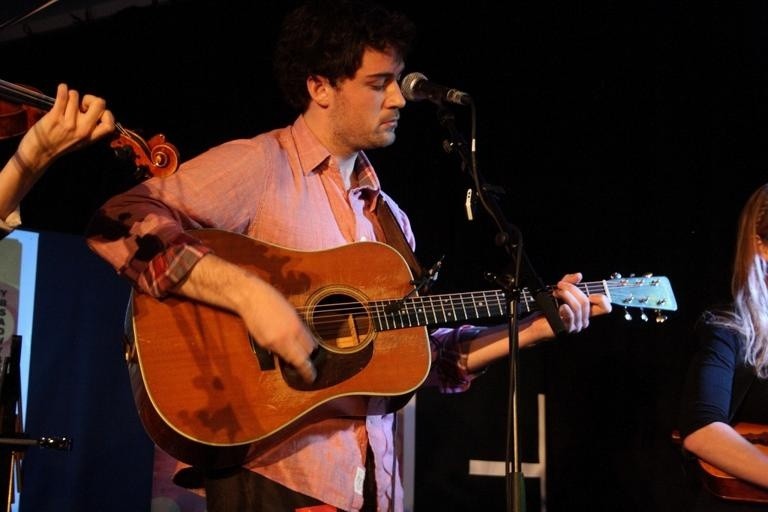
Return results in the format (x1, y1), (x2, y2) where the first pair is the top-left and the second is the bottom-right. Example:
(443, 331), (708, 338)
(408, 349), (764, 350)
(83, 24), (613, 512)
(675, 183), (768, 512)
(1, 83), (116, 238)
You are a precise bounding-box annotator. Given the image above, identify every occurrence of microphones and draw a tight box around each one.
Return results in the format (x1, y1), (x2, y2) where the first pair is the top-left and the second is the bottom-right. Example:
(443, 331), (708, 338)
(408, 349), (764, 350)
(401, 70), (469, 106)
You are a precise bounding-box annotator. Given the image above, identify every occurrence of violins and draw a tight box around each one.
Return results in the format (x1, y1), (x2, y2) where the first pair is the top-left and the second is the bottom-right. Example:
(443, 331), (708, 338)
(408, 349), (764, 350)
(0, 77), (180, 178)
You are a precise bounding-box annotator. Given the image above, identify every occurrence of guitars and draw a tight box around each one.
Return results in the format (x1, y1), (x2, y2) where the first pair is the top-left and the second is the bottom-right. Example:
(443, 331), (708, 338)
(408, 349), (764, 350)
(123, 226), (678, 467)
(698, 423), (766, 503)
